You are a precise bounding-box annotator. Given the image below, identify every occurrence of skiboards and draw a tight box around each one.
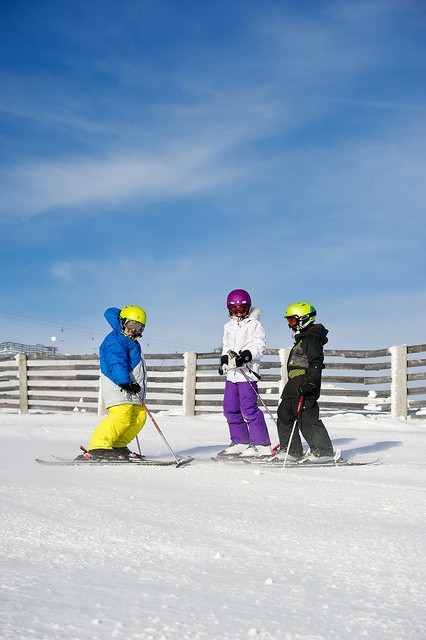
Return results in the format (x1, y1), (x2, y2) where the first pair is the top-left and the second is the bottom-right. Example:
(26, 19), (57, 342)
(210, 456), (274, 462)
(244, 459), (377, 468)
(35, 454), (195, 466)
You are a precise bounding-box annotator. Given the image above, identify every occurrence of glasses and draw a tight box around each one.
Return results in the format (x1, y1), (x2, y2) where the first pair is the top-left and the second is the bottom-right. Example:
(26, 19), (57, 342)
(124, 320), (145, 335)
(229, 306), (247, 313)
(286, 317), (298, 327)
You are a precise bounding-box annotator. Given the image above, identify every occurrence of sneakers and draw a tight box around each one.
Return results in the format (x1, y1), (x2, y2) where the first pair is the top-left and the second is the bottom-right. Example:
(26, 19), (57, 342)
(113, 448), (140, 458)
(298, 453), (335, 464)
(274, 447), (302, 462)
(239, 445), (272, 457)
(223, 443), (249, 454)
(89, 450), (128, 460)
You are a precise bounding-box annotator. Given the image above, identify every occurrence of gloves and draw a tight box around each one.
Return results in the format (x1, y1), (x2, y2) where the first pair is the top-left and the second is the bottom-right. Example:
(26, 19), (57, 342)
(299, 381), (319, 397)
(236, 350), (252, 367)
(118, 383), (140, 393)
(219, 355), (228, 375)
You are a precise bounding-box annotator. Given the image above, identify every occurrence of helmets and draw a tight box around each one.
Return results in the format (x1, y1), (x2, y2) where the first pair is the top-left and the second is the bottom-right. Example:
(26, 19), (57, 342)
(284, 302), (317, 332)
(227, 289), (251, 319)
(118, 305), (146, 342)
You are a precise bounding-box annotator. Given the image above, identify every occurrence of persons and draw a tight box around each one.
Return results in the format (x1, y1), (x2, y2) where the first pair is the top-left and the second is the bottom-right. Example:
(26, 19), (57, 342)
(274, 302), (335, 462)
(219, 290), (271, 459)
(87, 305), (148, 460)
